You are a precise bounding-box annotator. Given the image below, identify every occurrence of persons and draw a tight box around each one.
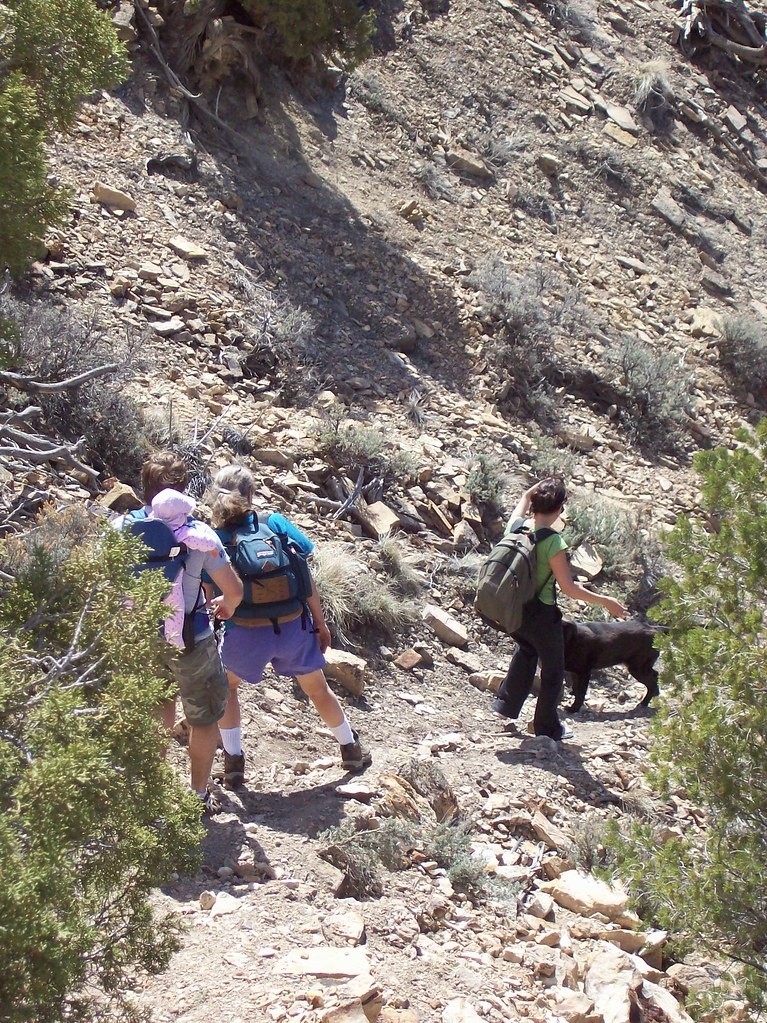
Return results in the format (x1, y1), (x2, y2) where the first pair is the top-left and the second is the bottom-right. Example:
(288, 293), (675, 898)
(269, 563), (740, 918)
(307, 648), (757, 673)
(492, 476), (631, 741)
(202, 466), (372, 783)
(101, 452), (243, 816)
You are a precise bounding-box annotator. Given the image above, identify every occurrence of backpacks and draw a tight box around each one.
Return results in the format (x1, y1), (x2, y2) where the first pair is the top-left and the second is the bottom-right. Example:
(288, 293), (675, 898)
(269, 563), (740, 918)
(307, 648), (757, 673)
(125, 509), (191, 598)
(215, 513), (311, 606)
(473, 526), (560, 633)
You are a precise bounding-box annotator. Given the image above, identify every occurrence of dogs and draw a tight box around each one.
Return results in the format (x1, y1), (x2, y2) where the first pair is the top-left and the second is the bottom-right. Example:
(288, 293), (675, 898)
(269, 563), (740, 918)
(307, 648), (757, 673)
(562, 619), (661, 714)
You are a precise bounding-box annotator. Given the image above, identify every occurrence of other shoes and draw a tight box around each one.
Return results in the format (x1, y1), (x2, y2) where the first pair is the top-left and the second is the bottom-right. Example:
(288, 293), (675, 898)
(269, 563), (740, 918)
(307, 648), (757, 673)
(197, 788), (222, 815)
(492, 707), (506, 720)
(560, 722), (567, 738)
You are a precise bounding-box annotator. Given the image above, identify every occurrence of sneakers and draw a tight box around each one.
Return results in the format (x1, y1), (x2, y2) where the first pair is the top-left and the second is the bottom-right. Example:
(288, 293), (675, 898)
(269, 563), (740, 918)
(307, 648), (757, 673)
(340, 730), (372, 770)
(222, 747), (246, 789)
